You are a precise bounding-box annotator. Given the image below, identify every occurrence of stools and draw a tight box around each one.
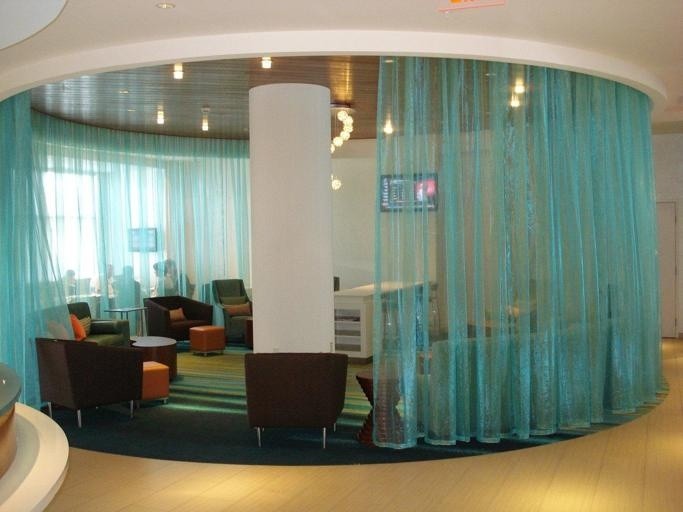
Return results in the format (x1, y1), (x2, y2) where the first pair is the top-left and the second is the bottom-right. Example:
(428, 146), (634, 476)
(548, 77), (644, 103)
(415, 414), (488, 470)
(190, 325), (226, 357)
(140, 361), (170, 406)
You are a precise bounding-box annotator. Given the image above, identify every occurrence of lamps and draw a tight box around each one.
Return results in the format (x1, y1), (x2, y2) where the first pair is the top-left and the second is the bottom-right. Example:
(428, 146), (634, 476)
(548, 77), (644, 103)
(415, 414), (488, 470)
(330, 103), (357, 189)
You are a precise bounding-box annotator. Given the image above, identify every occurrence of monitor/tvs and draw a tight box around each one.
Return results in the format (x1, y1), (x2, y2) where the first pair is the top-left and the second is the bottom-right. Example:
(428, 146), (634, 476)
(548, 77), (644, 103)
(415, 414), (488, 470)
(128, 228), (157, 252)
(380, 173), (439, 212)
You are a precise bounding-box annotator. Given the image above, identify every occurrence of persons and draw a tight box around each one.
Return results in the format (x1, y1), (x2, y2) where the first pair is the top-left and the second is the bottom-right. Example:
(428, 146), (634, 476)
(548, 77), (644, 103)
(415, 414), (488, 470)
(111, 265), (141, 321)
(55, 270), (77, 296)
(94, 264), (115, 319)
(165, 258), (191, 298)
(153, 261), (176, 297)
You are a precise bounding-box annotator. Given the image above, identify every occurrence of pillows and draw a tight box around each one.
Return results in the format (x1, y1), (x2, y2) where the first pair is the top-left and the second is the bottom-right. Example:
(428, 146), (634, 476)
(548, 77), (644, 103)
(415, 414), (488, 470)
(169, 307), (185, 322)
(221, 297), (250, 316)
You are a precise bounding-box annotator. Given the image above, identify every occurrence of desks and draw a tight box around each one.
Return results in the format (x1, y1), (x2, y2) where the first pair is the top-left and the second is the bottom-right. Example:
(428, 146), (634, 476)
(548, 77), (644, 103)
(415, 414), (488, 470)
(104, 306), (149, 337)
(334, 280), (428, 361)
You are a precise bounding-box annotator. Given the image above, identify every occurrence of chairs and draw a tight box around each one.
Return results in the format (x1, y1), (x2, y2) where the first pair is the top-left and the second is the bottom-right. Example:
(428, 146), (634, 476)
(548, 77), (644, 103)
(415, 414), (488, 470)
(211, 278), (253, 342)
(244, 352), (348, 451)
(25, 300), (142, 429)
(143, 295), (213, 342)
